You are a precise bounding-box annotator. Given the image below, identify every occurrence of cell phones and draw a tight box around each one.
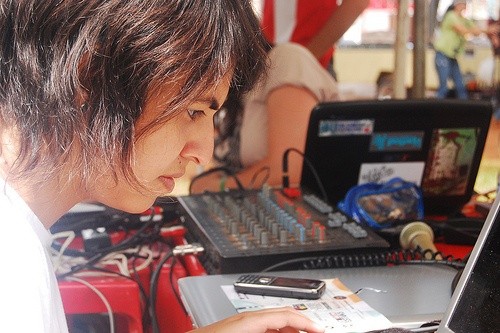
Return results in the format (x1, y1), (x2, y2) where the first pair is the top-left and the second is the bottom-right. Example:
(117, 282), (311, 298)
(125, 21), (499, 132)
(233, 274), (326, 299)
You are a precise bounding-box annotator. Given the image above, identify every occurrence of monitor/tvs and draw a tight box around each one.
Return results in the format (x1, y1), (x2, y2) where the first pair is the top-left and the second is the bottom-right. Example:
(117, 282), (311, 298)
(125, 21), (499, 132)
(301, 99), (493, 214)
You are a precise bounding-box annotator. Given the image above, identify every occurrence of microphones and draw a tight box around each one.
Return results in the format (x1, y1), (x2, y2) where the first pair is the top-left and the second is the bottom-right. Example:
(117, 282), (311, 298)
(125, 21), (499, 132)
(395, 221), (444, 261)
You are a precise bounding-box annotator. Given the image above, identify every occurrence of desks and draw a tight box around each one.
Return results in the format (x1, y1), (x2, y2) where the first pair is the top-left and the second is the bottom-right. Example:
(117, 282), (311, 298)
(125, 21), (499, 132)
(176, 261), (456, 333)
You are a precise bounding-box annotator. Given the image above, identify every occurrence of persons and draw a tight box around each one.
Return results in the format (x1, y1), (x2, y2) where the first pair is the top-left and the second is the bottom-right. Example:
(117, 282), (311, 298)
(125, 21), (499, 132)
(431, 0), (481, 99)
(186, 36), (341, 195)
(0, 0), (325, 333)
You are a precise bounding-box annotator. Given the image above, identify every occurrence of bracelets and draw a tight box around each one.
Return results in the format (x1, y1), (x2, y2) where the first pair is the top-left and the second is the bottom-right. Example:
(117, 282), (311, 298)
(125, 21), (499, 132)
(220, 176), (230, 193)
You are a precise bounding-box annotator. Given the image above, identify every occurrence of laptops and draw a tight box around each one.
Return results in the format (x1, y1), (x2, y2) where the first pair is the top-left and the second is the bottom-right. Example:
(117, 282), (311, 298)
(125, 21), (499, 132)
(354, 187), (500, 333)
